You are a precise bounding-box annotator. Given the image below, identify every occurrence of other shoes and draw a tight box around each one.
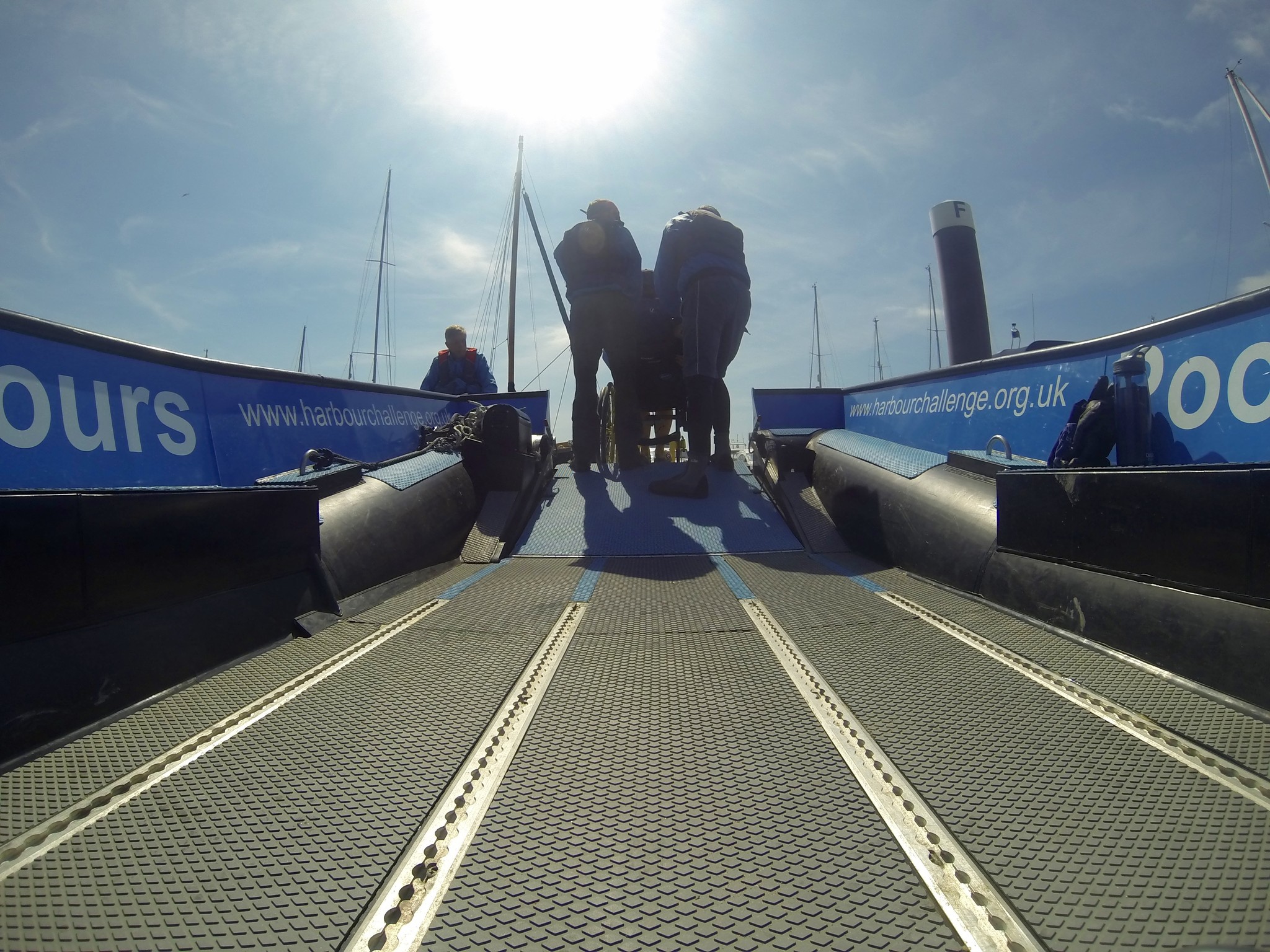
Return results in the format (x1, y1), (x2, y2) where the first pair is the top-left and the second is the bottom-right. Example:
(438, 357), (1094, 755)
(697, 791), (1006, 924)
(654, 450), (672, 464)
(710, 454), (734, 472)
(568, 458), (591, 471)
(639, 454), (651, 464)
(620, 455), (643, 471)
(648, 472), (709, 499)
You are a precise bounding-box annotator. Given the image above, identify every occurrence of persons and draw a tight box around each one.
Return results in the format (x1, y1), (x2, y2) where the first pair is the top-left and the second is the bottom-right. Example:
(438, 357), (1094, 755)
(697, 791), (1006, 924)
(551, 199), (649, 471)
(419, 325), (498, 394)
(635, 268), (682, 462)
(642, 204), (752, 497)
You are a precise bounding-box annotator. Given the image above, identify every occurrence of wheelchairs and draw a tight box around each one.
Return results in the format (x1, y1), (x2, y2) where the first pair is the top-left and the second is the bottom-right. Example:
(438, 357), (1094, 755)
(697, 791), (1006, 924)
(598, 356), (688, 481)
